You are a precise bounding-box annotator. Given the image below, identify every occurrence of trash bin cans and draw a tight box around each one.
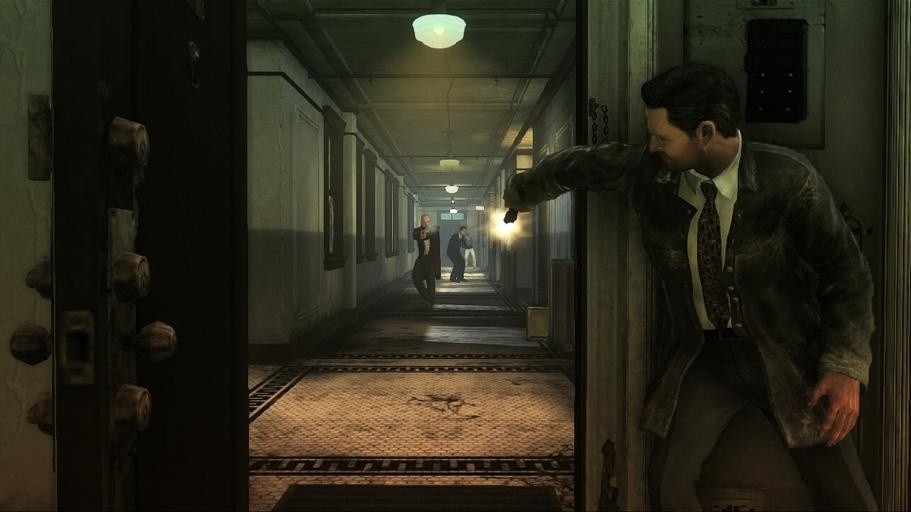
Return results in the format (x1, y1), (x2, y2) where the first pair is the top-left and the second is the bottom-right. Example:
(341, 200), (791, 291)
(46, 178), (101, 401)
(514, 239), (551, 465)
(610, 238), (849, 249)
(527, 307), (550, 338)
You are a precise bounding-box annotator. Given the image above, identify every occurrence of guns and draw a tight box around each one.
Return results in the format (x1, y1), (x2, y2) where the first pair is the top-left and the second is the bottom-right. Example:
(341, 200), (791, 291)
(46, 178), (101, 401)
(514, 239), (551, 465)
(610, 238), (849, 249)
(420, 225), (427, 231)
(503, 209), (520, 224)
